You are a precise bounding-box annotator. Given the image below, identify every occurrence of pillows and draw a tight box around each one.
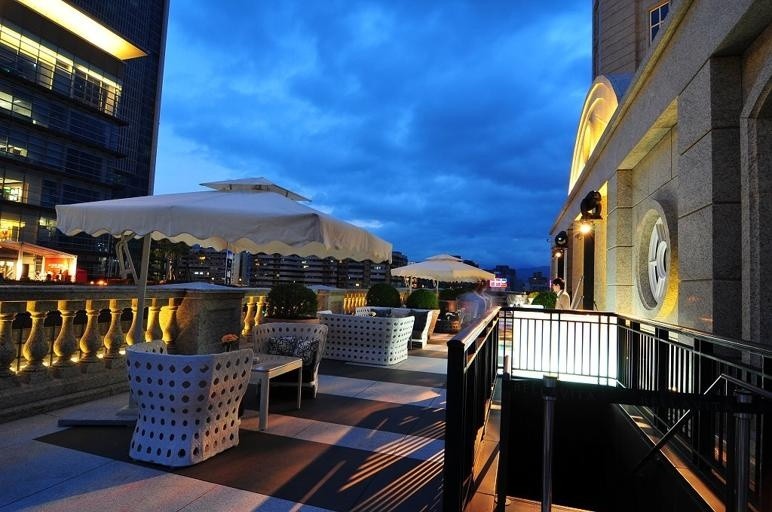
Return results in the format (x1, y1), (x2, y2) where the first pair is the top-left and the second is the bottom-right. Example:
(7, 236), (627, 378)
(268, 336), (299, 356)
(409, 311), (428, 330)
(370, 307), (391, 317)
(293, 336), (320, 365)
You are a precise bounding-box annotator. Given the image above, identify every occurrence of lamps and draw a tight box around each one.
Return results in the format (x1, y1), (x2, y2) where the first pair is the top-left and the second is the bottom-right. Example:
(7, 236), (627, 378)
(553, 230), (569, 257)
(578, 190), (602, 235)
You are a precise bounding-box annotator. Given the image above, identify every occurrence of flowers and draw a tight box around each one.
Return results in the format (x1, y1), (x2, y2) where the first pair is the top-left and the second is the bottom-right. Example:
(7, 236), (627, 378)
(368, 312), (377, 316)
(221, 333), (239, 343)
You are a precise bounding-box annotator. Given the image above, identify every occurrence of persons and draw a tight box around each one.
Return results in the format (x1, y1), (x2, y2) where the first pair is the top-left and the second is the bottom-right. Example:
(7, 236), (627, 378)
(553, 278), (570, 310)
(458, 279), (492, 329)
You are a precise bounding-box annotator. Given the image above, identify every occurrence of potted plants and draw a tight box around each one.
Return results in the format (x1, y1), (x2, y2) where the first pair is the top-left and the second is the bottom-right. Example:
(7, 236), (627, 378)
(264, 283), (320, 324)
(406, 288), (441, 339)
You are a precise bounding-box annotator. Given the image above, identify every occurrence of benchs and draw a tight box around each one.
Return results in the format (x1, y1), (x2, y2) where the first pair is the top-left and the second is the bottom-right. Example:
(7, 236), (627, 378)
(316, 310), (415, 366)
(355, 306), (432, 350)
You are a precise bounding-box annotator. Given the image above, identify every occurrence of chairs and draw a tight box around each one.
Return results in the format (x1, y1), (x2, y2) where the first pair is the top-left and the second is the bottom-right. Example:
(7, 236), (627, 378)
(252, 322), (328, 399)
(125, 339), (254, 467)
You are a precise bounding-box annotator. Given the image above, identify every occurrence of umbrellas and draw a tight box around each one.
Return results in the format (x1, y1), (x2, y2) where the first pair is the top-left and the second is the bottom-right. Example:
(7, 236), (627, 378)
(55, 177), (393, 410)
(391, 253), (495, 297)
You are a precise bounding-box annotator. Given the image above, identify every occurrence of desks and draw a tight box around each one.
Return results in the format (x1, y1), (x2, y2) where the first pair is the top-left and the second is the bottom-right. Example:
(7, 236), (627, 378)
(247, 352), (303, 433)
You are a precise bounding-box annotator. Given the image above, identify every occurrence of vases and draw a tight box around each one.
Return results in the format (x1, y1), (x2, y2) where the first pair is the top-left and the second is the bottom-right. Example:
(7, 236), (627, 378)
(228, 343), (236, 352)
